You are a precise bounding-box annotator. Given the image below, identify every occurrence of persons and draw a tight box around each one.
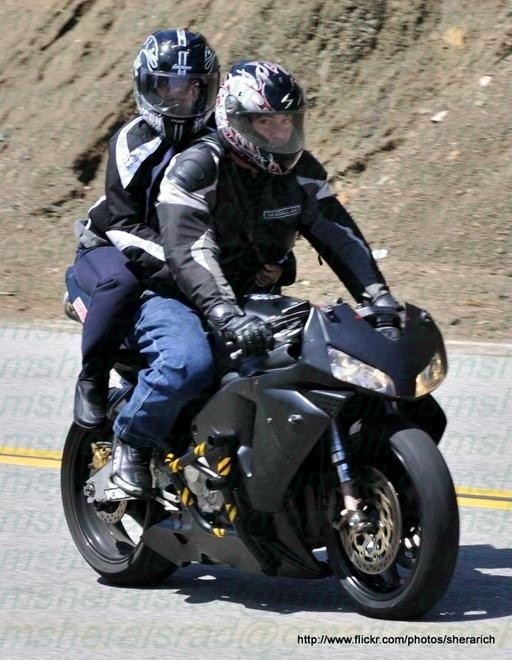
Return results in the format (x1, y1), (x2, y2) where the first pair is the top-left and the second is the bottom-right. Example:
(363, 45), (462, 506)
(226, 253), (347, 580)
(72, 26), (298, 433)
(108, 59), (405, 502)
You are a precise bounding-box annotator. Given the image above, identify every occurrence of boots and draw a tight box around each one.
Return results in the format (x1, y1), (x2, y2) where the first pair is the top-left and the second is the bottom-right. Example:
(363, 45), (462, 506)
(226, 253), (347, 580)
(74, 353), (109, 433)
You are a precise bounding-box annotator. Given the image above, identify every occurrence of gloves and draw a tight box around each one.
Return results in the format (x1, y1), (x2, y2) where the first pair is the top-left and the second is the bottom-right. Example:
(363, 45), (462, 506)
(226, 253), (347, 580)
(201, 298), (275, 356)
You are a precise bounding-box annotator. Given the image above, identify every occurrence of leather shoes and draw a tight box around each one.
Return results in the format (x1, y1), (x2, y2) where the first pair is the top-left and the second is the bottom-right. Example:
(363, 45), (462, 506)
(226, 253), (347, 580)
(111, 432), (152, 498)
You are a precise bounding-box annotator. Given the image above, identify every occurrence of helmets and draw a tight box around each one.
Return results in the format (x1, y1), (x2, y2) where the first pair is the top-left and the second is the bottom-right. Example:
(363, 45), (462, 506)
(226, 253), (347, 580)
(132, 28), (220, 144)
(214, 61), (307, 177)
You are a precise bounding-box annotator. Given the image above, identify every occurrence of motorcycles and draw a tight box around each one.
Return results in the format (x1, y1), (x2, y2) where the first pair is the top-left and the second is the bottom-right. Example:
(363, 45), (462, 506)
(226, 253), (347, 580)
(58, 285), (463, 621)
